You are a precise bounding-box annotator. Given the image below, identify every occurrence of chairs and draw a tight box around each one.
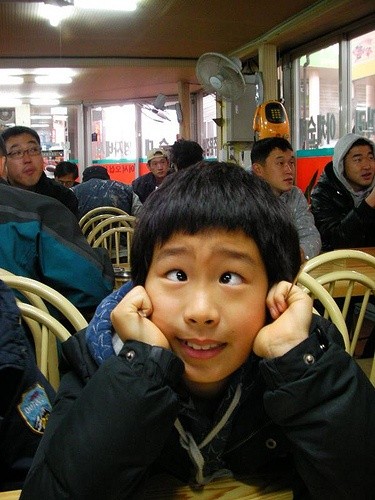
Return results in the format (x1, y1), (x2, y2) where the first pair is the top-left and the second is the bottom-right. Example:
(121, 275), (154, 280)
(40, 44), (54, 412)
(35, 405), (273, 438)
(78, 206), (138, 291)
(0, 268), (90, 393)
(295, 250), (375, 387)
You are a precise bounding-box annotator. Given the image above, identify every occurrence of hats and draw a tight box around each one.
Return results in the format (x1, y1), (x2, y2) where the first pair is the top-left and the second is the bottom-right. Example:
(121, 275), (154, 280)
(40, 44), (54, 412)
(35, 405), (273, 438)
(147, 149), (169, 160)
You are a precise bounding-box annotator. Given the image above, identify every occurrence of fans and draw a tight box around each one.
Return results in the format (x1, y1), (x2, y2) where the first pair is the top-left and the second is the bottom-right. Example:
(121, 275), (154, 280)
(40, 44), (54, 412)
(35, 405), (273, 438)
(196, 53), (264, 106)
(135, 94), (183, 124)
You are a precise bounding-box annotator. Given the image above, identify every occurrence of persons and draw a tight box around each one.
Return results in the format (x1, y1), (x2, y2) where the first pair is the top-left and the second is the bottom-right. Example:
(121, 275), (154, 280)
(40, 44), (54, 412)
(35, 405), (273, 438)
(310, 134), (375, 358)
(169, 139), (203, 170)
(68, 166), (145, 264)
(18, 159), (375, 499)
(131, 148), (175, 207)
(1, 127), (81, 218)
(0, 133), (116, 381)
(243, 138), (320, 265)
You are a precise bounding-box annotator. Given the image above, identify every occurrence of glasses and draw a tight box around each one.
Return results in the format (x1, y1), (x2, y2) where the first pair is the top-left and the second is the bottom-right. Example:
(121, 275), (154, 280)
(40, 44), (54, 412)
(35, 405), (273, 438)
(5, 146), (42, 158)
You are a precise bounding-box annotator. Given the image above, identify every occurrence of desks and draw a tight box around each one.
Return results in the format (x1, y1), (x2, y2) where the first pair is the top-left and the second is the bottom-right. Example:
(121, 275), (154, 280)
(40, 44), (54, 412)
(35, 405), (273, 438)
(294, 247), (375, 299)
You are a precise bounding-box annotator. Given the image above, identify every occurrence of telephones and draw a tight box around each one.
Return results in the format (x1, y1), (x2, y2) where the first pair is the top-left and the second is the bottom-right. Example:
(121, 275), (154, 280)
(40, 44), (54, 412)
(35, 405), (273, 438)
(253, 100), (291, 145)
